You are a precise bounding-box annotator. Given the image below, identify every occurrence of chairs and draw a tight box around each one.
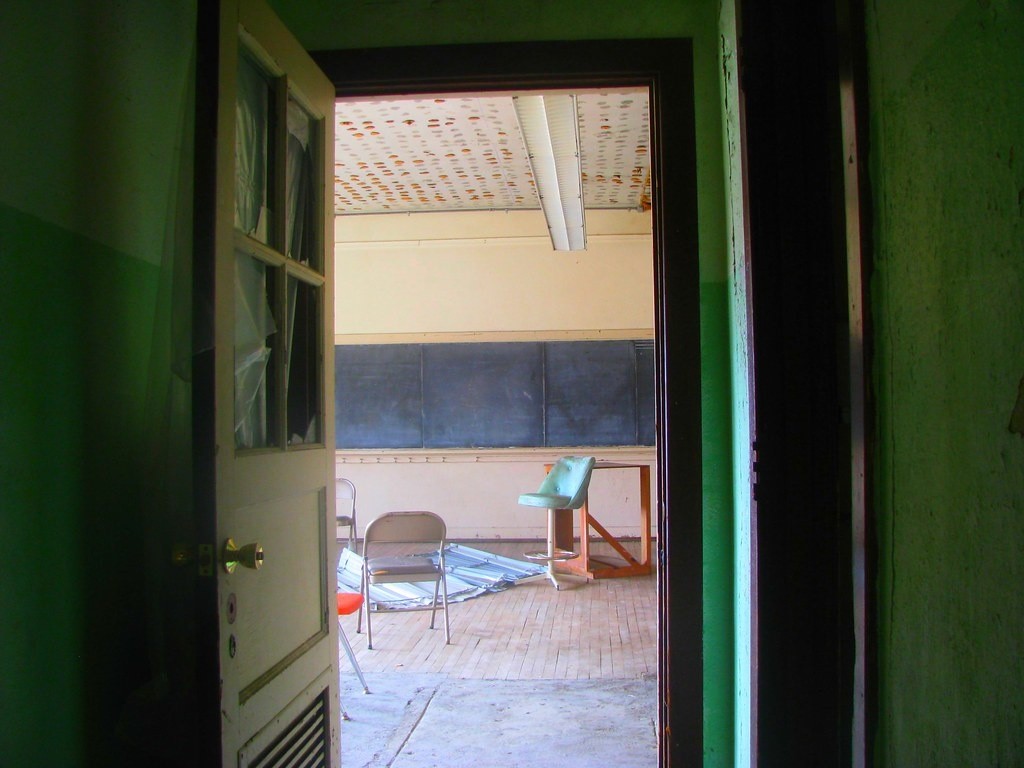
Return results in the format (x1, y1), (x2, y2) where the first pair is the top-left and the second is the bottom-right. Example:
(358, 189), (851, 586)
(356, 510), (450, 649)
(335, 478), (358, 554)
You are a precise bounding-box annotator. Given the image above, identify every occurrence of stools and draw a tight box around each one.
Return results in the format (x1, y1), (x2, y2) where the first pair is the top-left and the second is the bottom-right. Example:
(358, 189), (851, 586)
(512, 456), (596, 591)
(336, 592), (372, 720)
